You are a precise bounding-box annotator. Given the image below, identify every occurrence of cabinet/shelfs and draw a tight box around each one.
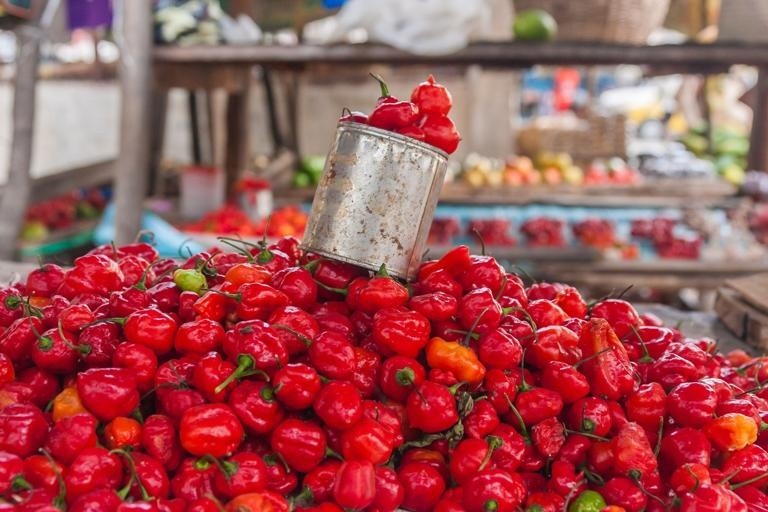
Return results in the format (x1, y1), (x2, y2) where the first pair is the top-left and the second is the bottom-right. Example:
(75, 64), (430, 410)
(137, 43), (767, 298)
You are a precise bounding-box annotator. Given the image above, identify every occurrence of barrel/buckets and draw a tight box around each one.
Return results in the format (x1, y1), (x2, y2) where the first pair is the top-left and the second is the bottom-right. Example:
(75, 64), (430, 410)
(296, 120), (449, 283)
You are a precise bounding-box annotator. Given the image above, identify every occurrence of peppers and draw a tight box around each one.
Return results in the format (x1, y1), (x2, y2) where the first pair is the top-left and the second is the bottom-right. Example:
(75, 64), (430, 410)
(0, 225), (768, 512)
(340, 72), (464, 156)
